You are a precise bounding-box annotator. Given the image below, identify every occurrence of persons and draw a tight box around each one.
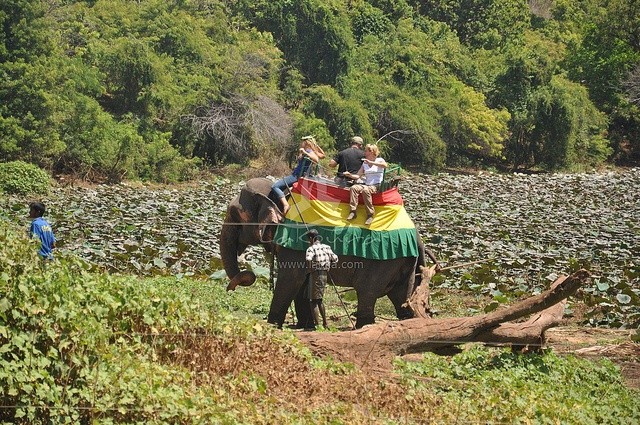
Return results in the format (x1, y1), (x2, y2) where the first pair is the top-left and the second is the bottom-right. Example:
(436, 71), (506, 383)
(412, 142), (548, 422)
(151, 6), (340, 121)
(272, 136), (325, 213)
(28, 201), (56, 263)
(305, 230), (338, 331)
(347, 144), (388, 225)
(329, 136), (366, 181)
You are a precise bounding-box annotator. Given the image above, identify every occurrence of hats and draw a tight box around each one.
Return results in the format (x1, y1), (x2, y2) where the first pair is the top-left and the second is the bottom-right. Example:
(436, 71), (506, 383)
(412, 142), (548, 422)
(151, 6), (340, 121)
(351, 136), (364, 147)
(309, 229), (319, 237)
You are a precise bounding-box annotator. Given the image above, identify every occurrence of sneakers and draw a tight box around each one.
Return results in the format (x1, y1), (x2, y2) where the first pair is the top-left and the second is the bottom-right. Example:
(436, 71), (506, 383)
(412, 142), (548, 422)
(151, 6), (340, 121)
(364, 215), (372, 225)
(347, 212), (357, 220)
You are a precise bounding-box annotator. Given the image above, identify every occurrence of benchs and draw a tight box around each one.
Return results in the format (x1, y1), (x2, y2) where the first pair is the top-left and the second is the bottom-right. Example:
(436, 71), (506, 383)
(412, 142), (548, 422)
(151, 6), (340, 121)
(299, 156), (400, 204)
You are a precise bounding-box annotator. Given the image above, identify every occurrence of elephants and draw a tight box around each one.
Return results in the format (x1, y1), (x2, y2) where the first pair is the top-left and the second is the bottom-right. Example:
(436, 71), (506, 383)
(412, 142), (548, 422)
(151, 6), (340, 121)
(219, 177), (428, 332)
